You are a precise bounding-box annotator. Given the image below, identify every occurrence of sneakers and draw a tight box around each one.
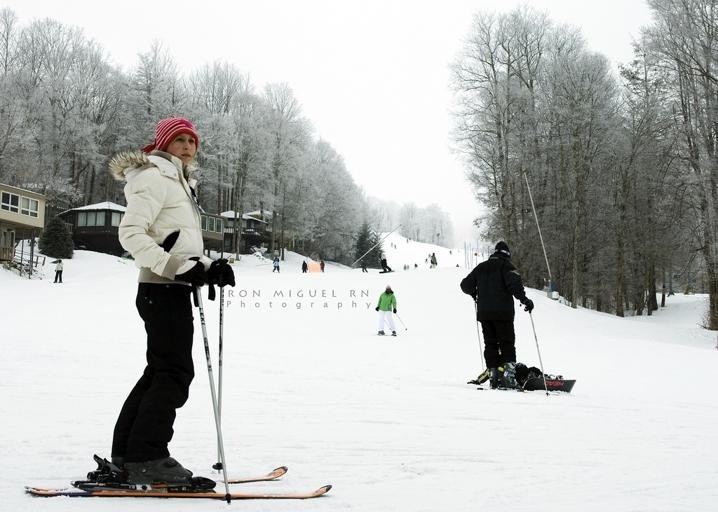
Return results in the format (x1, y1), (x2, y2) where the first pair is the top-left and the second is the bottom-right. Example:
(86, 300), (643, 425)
(111, 454), (192, 482)
(489, 368), (497, 381)
(501, 366), (515, 383)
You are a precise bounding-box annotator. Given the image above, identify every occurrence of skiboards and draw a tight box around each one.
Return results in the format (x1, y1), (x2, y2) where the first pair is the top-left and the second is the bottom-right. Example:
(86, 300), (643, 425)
(26, 468), (333, 498)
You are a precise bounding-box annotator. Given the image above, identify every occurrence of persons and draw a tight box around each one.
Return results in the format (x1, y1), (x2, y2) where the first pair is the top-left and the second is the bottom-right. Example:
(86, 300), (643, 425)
(378, 249), (391, 273)
(111, 118), (235, 484)
(320, 260), (324, 272)
(302, 261), (307, 273)
(360, 258), (368, 272)
(429, 253), (437, 268)
(273, 256), (280, 272)
(53, 259), (64, 283)
(375, 285), (398, 336)
(461, 242), (534, 368)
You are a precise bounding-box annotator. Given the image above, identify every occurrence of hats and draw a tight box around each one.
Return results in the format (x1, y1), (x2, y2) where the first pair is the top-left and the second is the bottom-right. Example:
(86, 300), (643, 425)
(495, 241), (511, 257)
(386, 285), (391, 290)
(155, 117), (199, 153)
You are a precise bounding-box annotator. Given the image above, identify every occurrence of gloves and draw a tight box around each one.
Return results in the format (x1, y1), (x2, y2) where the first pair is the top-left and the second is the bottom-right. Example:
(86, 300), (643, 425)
(177, 256), (235, 286)
(521, 297), (533, 311)
